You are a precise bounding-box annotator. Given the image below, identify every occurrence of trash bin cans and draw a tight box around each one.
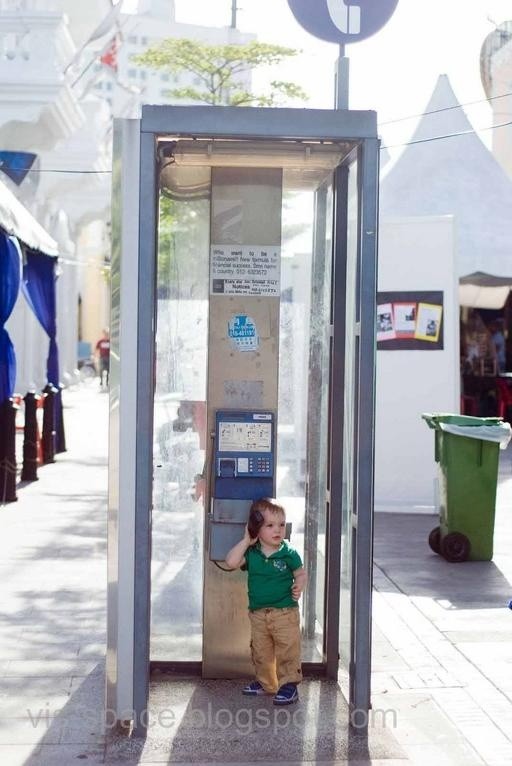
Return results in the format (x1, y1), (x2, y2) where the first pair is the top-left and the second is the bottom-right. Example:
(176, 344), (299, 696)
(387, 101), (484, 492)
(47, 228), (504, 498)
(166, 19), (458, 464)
(421, 412), (505, 562)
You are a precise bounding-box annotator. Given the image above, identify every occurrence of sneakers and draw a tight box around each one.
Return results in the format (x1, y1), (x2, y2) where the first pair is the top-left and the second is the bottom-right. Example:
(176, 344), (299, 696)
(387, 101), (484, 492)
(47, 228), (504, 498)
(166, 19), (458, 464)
(272, 683), (298, 705)
(240, 681), (267, 695)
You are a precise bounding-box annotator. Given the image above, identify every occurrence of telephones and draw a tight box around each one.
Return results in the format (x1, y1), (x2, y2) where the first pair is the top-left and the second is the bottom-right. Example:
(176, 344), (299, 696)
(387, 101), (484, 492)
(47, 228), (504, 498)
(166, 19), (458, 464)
(248, 511), (265, 539)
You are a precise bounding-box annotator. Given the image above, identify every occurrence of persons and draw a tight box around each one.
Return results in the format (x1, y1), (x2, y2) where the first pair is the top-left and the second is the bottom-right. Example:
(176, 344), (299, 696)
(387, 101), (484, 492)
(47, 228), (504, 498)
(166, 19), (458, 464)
(242, 498), (307, 706)
